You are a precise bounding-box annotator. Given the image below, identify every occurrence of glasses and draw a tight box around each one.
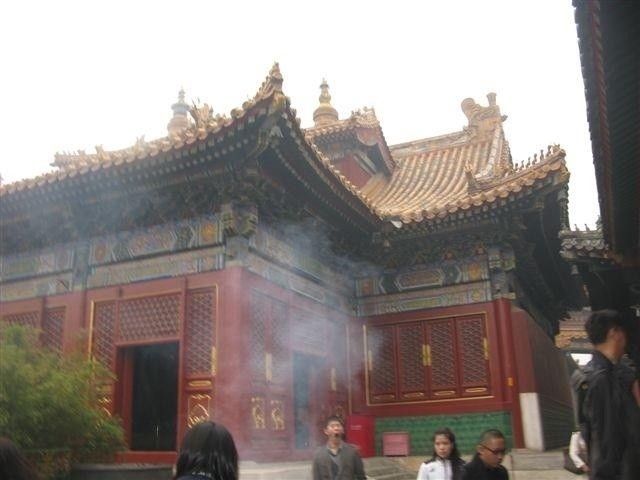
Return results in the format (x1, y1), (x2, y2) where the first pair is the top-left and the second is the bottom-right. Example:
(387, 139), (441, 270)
(480, 444), (505, 454)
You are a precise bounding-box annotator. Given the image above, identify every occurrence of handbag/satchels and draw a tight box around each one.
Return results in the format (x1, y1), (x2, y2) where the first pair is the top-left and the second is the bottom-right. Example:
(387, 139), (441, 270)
(562, 449), (585, 475)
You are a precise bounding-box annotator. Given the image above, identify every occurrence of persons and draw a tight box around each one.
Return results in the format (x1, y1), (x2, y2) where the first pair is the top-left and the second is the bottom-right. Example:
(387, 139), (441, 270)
(416, 427), (469, 480)
(452, 428), (509, 480)
(568, 426), (591, 474)
(312, 414), (367, 480)
(0, 435), (47, 480)
(170, 419), (241, 480)
(568, 307), (639, 480)
